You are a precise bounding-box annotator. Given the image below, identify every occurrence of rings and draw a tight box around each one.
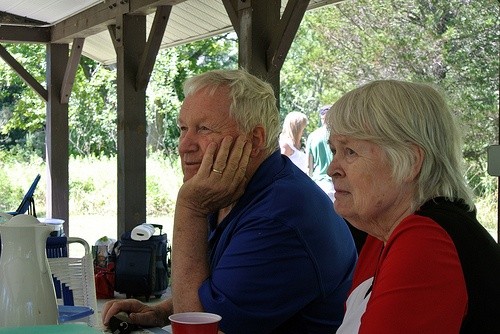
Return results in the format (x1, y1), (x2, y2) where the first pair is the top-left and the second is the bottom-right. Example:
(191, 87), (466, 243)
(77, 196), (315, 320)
(212, 169), (222, 174)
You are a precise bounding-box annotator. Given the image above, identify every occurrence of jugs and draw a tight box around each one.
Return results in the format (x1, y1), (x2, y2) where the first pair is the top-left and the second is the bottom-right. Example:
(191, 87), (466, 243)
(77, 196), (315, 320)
(0, 214), (59, 325)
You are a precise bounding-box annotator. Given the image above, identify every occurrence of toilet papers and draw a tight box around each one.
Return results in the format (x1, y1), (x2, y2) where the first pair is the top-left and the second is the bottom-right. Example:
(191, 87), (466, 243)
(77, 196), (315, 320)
(131, 223), (155, 241)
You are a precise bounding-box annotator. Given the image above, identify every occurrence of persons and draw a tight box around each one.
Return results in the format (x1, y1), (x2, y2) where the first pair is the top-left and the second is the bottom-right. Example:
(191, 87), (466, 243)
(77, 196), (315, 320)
(307, 105), (336, 204)
(279, 111), (309, 176)
(326, 81), (500, 334)
(100, 68), (359, 334)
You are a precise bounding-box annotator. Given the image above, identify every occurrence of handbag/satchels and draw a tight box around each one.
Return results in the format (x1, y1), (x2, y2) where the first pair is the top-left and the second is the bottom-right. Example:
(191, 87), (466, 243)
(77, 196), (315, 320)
(115, 224), (168, 299)
(95, 262), (114, 298)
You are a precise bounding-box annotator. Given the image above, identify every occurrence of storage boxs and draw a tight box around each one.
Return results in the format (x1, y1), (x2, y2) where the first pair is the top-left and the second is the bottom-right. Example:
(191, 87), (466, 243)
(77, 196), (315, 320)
(58, 304), (95, 327)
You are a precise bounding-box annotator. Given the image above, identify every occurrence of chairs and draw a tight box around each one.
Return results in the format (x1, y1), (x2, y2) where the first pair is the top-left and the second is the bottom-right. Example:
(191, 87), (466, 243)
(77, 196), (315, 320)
(46, 237), (98, 328)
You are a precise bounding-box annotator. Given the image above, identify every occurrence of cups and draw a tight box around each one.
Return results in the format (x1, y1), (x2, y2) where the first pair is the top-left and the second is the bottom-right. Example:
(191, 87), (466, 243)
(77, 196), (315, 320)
(168, 312), (222, 334)
